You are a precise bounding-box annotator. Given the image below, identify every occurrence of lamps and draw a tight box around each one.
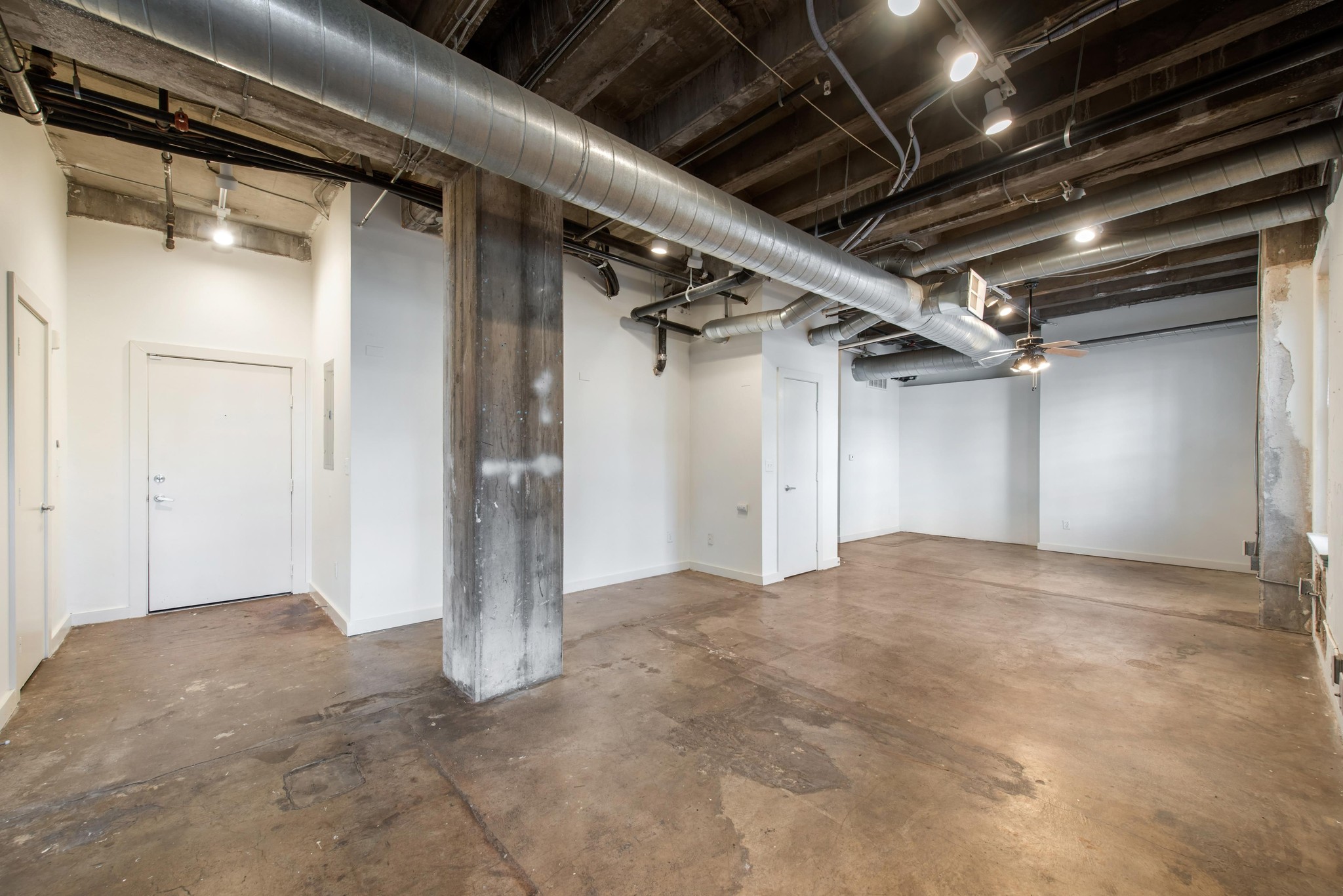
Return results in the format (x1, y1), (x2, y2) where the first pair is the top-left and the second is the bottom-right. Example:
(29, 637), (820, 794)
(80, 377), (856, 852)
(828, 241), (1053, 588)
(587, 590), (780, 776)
(650, 236), (669, 258)
(934, 15), (983, 86)
(1010, 347), (1053, 392)
(986, 289), (1001, 311)
(687, 247), (704, 269)
(208, 205), (236, 247)
(980, 85), (1015, 138)
(1060, 179), (1085, 202)
(997, 298), (1015, 320)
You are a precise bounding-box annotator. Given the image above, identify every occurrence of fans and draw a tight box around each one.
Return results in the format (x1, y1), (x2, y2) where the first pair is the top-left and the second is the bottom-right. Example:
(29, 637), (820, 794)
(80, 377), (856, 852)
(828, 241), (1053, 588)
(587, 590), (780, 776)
(974, 281), (1091, 372)
